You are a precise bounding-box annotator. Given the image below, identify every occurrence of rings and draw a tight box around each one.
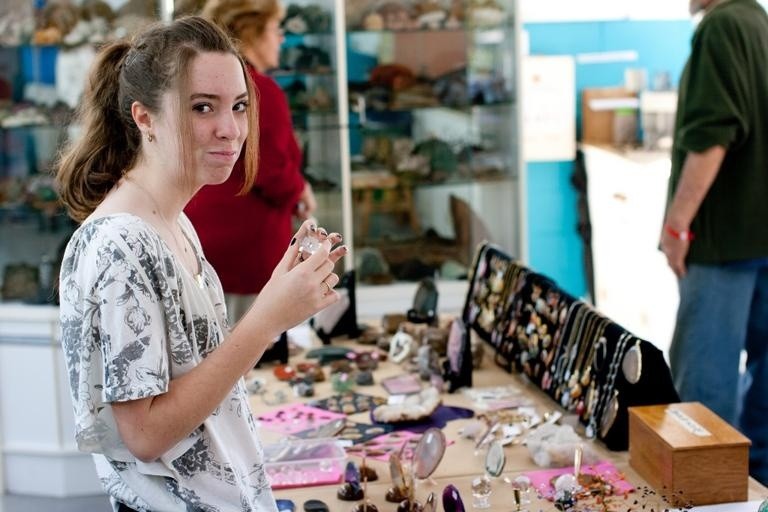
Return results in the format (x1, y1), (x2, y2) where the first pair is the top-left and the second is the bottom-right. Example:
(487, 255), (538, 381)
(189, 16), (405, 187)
(299, 251), (305, 262)
(323, 281), (332, 292)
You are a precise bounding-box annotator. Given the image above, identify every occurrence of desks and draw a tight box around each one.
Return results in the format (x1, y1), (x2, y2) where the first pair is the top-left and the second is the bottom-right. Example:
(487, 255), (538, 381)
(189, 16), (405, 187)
(248, 320), (768, 512)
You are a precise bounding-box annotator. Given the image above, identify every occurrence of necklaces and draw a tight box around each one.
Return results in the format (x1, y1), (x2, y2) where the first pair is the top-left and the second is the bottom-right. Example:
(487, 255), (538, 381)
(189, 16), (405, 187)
(124, 169), (205, 288)
(542, 299), (638, 441)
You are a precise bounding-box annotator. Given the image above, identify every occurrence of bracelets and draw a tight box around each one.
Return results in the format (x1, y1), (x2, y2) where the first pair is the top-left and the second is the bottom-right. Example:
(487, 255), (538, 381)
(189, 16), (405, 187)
(664, 225), (694, 242)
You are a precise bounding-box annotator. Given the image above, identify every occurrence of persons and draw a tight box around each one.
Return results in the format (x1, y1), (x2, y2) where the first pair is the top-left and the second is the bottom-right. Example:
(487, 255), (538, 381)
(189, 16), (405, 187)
(655, 0), (768, 485)
(48, 13), (350, 510)
(181, 1), (319, 360)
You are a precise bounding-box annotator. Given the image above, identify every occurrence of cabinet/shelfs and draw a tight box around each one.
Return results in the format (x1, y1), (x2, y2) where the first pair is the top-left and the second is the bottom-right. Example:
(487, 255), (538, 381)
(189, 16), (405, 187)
(0, 0), (528, 498)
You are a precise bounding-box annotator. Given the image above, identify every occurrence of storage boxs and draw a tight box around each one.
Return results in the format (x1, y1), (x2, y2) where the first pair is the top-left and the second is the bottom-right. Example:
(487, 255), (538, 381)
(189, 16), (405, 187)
(627, 401), (752, 508)
